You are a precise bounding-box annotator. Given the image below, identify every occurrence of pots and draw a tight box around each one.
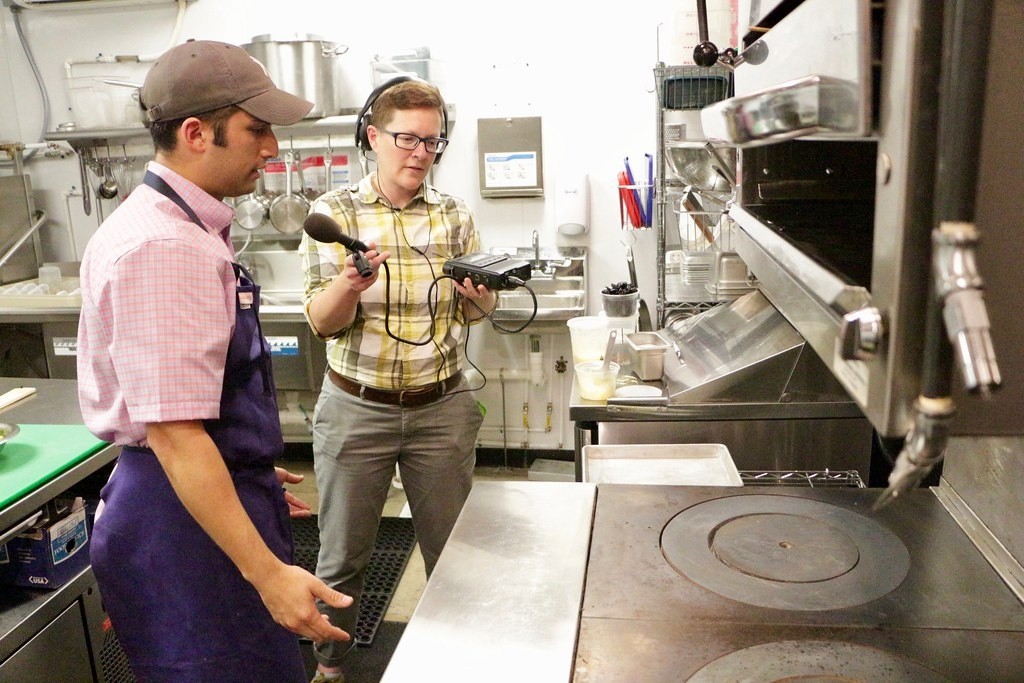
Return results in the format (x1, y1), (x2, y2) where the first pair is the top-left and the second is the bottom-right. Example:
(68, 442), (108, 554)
(371, 54), (445, 103)
(233, 149), (371, 235)
(236, 32), (350, 119)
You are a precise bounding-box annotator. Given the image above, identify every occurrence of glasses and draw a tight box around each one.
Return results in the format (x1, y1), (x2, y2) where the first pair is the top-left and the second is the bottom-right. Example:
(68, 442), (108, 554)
(374, 126), (450, 154)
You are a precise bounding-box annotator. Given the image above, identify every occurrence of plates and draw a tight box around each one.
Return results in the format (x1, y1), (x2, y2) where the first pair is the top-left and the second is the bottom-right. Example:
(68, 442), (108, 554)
(611, 384), (664, 400)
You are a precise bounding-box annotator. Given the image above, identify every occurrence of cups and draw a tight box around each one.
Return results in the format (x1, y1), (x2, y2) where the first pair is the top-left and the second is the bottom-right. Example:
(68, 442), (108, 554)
(38, 266), (62, 288)
(566, 316), (612, 368)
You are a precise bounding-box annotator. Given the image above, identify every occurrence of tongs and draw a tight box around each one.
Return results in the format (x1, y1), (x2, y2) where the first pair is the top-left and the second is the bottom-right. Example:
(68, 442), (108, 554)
(78, 149), (92, 217)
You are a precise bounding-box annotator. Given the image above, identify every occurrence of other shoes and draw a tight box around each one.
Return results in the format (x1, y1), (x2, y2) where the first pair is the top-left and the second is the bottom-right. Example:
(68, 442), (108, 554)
(310, 670), (344, 682)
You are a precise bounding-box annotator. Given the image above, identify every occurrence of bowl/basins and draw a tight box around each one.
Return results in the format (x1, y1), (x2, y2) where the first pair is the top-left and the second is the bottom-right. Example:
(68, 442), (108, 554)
(574, 360), (620, 400)
(664, 146), (737, 191)
(0, 423), (21, 452)
(601, 287), (641, 318)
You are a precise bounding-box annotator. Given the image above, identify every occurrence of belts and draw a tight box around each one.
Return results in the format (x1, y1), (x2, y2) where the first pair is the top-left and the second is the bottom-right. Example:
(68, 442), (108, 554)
(329, 365), (464, 412)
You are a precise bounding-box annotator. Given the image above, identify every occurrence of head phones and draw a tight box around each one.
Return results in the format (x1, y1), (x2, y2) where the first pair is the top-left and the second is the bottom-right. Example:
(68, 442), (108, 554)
(354, 76), (448, 164)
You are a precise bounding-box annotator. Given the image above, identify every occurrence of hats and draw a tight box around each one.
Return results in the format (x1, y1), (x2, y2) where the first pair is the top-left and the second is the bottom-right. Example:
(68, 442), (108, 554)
(139, 39), (316, 127)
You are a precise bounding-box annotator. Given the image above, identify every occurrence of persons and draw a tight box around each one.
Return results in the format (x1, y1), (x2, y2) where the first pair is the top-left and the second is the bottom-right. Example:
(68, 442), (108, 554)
(78, 39), (354, 683)
(300, 76), (500, 683)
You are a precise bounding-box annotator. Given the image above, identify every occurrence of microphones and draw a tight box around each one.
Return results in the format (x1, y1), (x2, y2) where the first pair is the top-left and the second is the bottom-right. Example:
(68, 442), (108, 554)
(304, 213), (386, 264)
